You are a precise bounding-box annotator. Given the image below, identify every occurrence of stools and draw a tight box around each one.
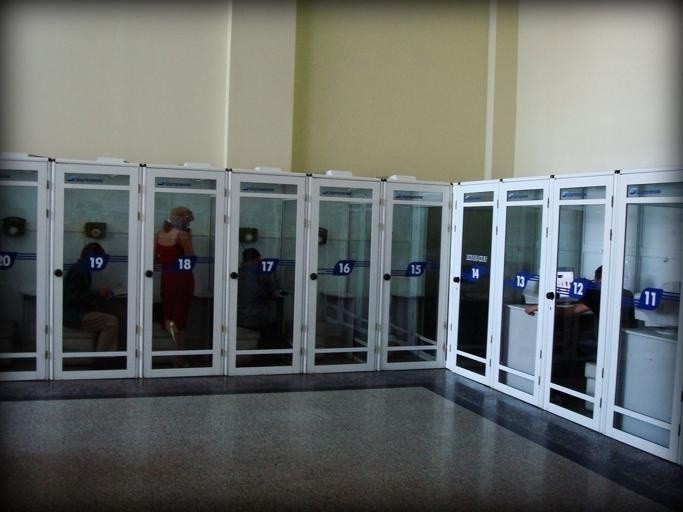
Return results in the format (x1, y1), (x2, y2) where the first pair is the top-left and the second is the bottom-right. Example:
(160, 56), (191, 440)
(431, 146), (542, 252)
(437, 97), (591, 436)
(584, 362), (597, 411)
(152, 325), (171, 364)
(0, 318), (22, 365)
(237, 327), (260, 365)
(316, 324), (344, 359)
(63, 329), (95, 365)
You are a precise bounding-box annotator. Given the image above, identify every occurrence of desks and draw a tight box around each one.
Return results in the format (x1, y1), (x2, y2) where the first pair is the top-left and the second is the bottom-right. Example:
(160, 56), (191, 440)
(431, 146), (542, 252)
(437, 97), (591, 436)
(614, 327), (678, 449)
(507, 302), (593, 396)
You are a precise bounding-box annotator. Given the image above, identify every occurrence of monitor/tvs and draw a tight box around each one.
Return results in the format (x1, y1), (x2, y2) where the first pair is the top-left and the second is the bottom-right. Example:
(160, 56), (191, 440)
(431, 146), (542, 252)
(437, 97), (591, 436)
(556, 267), (579, 308)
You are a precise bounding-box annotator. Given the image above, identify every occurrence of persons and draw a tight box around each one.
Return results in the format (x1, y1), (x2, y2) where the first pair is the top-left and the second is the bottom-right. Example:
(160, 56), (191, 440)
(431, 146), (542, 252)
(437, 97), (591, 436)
(64, 244), (120, 370)
(238, 249), (284, 350)
(155, 206), (196, 368)
(525, 265), (633, 390)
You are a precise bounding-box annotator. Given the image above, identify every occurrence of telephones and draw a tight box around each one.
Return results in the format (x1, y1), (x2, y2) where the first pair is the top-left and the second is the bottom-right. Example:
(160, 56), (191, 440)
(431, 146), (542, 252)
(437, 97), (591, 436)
(319, 228), (328, 246)
(1, 216), (27, 238)
